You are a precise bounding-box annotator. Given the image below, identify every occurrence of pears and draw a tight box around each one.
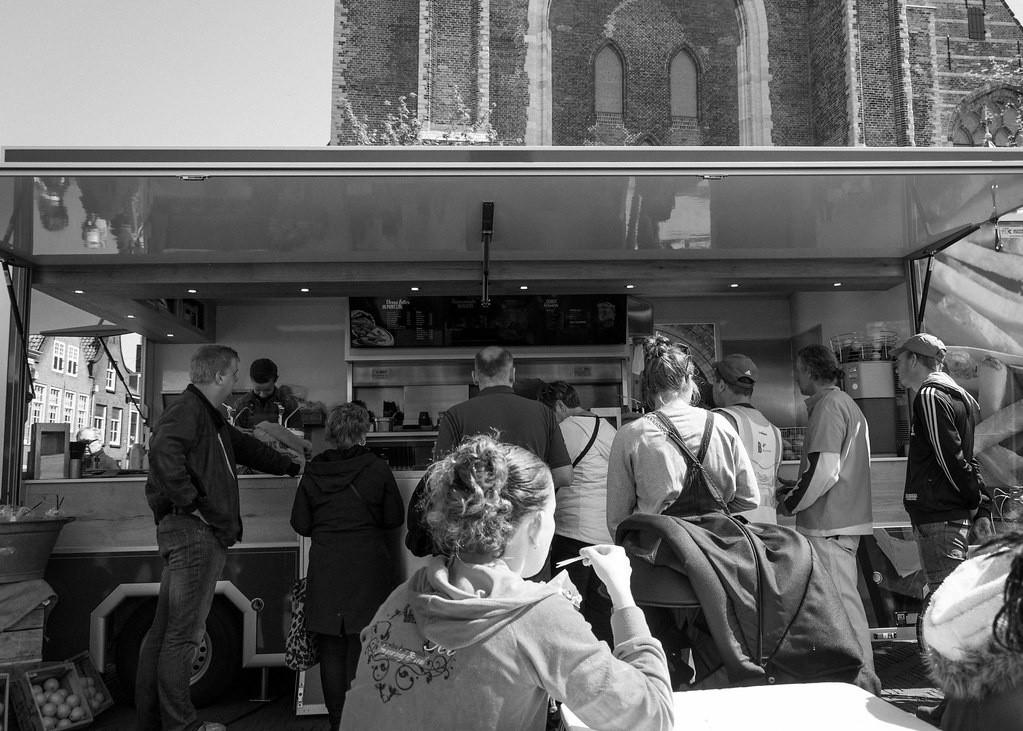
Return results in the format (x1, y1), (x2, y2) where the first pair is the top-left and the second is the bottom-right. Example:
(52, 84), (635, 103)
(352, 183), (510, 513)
(30, 676), (105, 731)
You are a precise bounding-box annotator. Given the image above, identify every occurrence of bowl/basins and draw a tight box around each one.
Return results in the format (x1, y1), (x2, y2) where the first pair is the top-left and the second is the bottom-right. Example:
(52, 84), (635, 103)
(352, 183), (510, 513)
(0, 516), (76, 583)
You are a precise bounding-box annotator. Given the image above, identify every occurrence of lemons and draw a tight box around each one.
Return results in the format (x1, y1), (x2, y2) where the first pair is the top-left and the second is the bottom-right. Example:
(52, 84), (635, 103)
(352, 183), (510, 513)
(837, 338), (883, 361)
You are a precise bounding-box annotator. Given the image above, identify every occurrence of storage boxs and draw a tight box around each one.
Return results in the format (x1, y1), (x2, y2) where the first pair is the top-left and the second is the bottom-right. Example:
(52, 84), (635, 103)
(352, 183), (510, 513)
(30, 422), (71, 480)
(65, 650), (116, 718)
(0, 673), (10, 731)
(0, 657), (43, 684)
(778, 426), (807, 461)
(9, 662), (94, 731)
(0, 601), (45, 664)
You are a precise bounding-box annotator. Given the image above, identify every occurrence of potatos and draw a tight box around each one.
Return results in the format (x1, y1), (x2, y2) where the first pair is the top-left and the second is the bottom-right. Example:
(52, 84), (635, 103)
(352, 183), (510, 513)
(781, 433), (804, 460)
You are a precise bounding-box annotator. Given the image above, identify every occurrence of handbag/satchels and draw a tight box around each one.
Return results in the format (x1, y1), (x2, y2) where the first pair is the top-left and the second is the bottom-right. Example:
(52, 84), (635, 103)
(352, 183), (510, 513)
(284, 576), (320, 672)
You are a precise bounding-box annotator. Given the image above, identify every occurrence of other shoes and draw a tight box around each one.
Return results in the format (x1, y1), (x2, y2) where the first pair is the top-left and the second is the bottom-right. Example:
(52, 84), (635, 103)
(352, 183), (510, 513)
(194, 720), (226, 731)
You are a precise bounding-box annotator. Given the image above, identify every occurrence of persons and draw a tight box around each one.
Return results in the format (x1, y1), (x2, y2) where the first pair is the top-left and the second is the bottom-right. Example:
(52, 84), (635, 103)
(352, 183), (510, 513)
(536, 380), (622, 653)
(289, 401), (405, 731)
(915, 526), (1023, 731)
(136, 345), (309, 730)
(700, 352), (781, 532)
(232, 356), (304, 434)
(434, 345), (574, 585)
(339, 427), (676, 731)
(78, 427), (121, 471)
(605, 331), (755, 689)
(887, 332), (983, 597)
(777, 344), (876, 673)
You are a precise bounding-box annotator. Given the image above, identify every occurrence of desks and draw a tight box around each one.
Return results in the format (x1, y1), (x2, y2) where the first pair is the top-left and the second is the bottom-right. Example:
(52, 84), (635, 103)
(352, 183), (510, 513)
(561, 680), (940, 731)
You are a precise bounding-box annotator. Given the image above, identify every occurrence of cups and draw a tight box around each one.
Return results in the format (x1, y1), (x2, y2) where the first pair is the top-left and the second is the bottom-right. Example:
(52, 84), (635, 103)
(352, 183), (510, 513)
(0, 504), (34, 522)
(42, 508), (65, 519)
(70, 459), (81, 479)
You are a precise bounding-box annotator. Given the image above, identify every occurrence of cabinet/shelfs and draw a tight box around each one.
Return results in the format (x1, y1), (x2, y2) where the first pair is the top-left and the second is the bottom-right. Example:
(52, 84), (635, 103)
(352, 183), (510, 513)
(57, 287), (217, 344)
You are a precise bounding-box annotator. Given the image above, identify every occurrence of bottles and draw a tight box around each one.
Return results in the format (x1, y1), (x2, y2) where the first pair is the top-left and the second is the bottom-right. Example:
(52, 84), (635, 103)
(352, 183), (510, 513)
(438, 413), (445, 431)
(131, 444), (141, 470)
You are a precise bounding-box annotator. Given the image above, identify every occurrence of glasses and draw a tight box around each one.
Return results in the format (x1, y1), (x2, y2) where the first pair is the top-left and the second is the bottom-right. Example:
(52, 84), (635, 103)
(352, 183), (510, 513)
(87, 438), (101, 446)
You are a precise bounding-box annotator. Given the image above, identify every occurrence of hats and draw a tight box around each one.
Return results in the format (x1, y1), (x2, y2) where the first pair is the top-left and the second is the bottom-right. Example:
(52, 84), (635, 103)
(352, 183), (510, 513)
(890, 333), (947, 364)
(711, 354), (758, 389)
